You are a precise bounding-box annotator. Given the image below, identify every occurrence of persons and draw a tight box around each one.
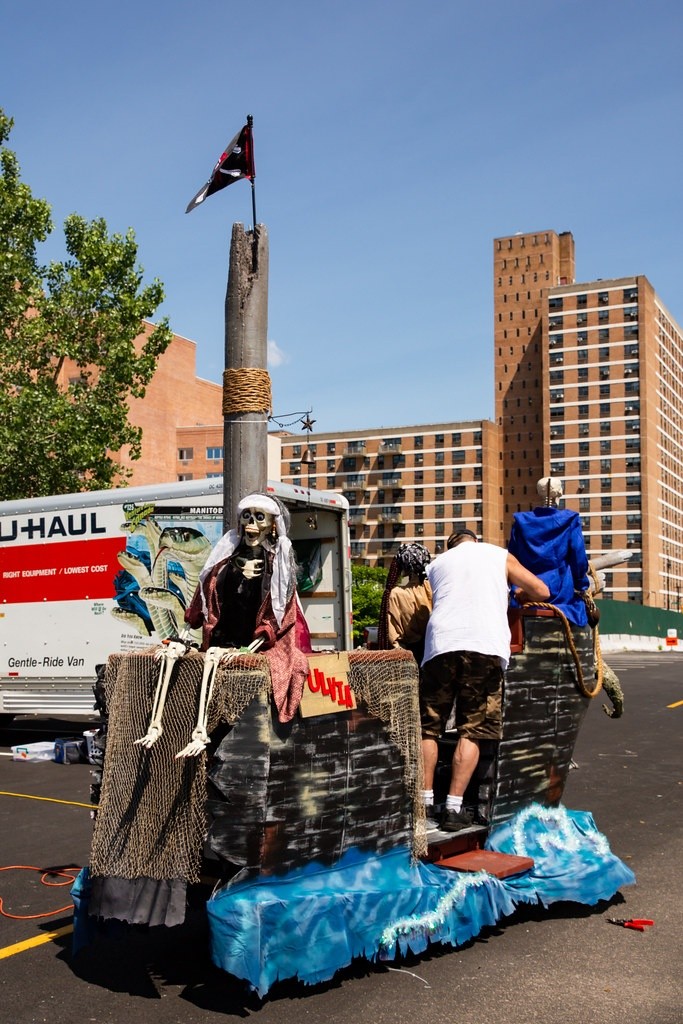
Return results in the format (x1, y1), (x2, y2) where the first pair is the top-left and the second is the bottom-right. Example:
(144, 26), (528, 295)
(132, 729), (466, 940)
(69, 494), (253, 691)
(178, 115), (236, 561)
(408, 530), (550, 833)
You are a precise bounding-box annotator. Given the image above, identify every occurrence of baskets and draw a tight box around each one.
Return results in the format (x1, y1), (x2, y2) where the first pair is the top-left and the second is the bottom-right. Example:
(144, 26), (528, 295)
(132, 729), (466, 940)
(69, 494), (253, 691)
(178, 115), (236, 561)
(84, 728), (104, 764)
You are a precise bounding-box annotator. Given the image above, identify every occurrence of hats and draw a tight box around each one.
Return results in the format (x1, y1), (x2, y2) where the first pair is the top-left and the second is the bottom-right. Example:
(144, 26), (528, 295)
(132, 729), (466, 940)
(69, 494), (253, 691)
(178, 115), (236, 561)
(447, 530), (477, 549)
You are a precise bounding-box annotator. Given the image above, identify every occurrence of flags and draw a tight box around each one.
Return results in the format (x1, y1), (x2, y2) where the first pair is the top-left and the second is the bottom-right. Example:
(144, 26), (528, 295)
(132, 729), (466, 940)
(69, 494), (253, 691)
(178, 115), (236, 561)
(183, 121), (252, 216)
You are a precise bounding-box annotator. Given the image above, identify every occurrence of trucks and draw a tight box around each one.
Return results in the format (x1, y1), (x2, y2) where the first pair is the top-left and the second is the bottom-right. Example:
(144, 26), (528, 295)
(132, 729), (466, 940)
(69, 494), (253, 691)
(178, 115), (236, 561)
(0, 476), (355, 732)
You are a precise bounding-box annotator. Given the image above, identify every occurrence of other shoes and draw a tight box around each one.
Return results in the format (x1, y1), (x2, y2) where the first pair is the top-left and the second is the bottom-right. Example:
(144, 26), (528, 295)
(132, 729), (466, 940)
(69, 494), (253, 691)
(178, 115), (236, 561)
(424, 806), (440, 820)
(443, 807), (472, 829)
